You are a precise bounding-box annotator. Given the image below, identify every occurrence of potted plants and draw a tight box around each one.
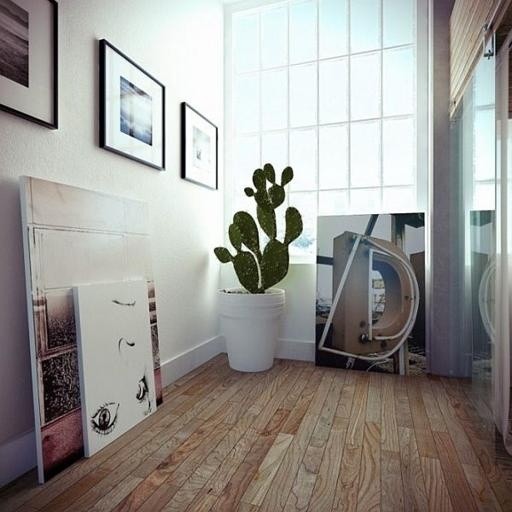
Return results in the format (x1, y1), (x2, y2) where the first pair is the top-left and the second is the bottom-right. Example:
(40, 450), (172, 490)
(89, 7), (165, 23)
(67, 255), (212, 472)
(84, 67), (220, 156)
(213, 162), (304, 373)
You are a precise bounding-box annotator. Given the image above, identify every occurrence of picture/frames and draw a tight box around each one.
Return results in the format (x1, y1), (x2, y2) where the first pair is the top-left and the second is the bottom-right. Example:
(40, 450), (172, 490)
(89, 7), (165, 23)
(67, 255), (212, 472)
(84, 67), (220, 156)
(181, 102), (218, 190)
(0, 0), (58, 130)
(99, 39), (165, 171)
